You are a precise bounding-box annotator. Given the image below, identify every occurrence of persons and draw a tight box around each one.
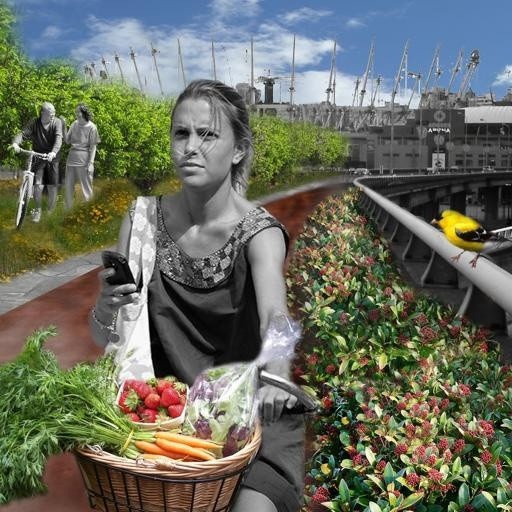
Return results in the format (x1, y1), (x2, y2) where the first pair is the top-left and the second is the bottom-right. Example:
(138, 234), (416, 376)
(85, 77), (310, 512)
(53, 105), (101, 209)
(8, 101), (64, 224)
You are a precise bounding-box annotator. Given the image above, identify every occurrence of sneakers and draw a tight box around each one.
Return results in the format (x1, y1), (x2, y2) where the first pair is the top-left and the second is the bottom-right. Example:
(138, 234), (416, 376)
(31, 207), (42, 224)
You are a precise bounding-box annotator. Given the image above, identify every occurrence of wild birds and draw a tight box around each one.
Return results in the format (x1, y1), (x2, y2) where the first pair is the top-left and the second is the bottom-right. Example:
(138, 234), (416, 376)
(430, 210), (512, 268)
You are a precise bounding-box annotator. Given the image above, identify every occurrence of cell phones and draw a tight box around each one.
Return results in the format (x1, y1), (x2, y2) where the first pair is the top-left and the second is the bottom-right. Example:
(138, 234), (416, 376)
(100, 249), (137, 295)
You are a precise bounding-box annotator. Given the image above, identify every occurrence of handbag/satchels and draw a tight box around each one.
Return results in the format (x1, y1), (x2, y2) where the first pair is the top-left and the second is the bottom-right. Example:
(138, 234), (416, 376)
(103, 196), (158, 389)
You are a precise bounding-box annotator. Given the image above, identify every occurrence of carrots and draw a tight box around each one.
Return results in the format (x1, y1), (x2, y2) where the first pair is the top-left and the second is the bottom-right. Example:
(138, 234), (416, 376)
(135, 432), (224, 462)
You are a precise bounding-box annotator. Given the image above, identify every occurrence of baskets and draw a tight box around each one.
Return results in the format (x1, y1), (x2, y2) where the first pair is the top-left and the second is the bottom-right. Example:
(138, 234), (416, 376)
(68, 416), (263, 512)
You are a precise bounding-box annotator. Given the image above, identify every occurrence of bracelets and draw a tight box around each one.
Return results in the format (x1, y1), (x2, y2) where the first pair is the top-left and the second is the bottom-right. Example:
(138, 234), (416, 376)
(91, 306), (118, 334)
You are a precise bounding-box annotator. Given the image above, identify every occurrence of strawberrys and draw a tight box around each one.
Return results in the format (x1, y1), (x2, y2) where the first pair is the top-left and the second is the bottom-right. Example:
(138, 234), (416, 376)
(118, 376), (187, 423)
